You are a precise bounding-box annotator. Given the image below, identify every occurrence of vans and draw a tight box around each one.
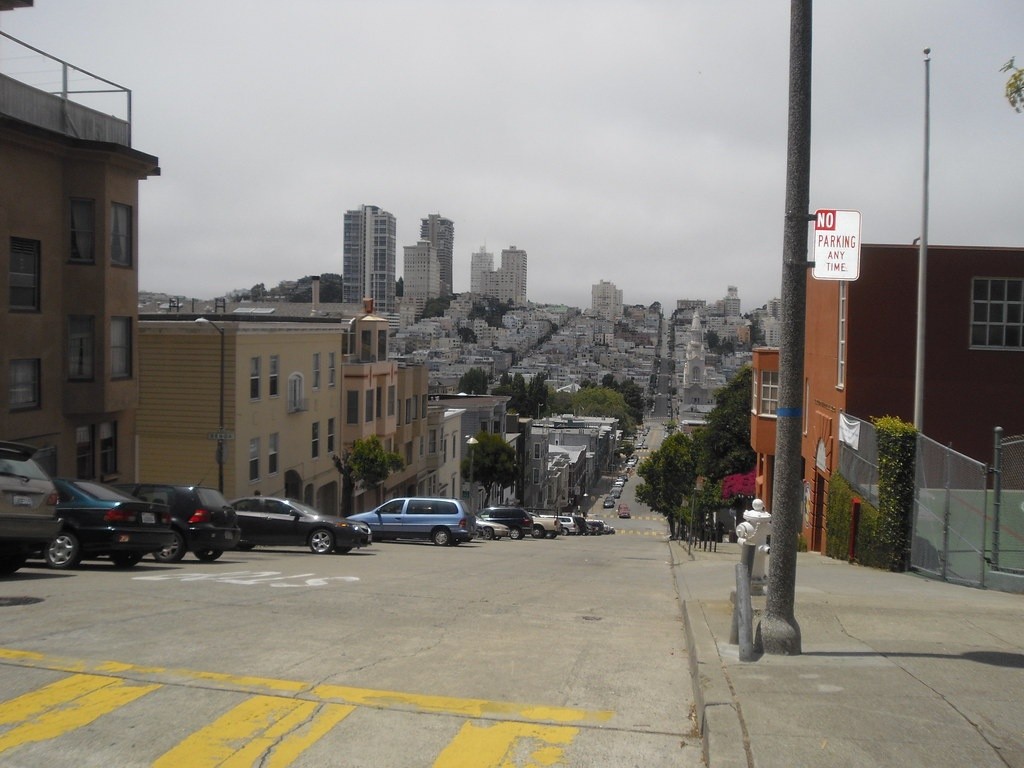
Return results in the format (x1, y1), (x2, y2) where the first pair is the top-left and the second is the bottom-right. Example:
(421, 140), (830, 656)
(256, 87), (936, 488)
(538, 515), (577, 536)
(571, 515), (588, 535)
(346, 497), (474, 547)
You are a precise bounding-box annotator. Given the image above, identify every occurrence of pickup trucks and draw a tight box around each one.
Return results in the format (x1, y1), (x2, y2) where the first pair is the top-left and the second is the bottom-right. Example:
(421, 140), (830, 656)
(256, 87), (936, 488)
(528, 511), (561, 539)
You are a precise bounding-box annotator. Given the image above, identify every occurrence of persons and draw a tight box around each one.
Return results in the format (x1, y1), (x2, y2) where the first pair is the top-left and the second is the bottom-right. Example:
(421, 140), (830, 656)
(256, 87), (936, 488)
(253, 490), (262, 497)
(715, 517), (725, 543)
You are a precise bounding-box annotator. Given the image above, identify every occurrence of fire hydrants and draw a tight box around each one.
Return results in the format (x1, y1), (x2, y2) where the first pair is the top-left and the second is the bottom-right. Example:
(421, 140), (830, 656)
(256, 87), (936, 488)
(736, 498), (771, 595)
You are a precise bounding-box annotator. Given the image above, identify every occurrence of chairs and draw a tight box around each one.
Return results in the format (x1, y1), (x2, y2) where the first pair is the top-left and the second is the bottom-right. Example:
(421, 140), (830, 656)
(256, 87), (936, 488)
(153, 498), (165, 504)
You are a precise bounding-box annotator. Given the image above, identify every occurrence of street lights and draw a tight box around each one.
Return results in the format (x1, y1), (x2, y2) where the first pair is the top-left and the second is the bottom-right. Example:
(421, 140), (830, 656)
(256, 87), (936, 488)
(466, 436), (479, 507)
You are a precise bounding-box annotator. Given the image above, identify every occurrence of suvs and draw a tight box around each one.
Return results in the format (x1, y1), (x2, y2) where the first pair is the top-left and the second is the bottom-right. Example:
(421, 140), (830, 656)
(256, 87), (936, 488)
(110, 483), (241, 564)
(0, 441), (63, 581)
(476, 507), (534, 540)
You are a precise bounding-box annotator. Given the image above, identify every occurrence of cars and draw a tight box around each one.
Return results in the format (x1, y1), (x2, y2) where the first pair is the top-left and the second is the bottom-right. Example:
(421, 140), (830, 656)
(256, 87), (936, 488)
(472, 513), (510, 541)
(585, 520), (615, 535)
(230, 494), (372, 554)
(28, 476), (174, 573)
(603, 424), (651, 508)
(619, 504), (631, 519)
(651, 359), (661, 413)
(664, 371), (673, 439)
